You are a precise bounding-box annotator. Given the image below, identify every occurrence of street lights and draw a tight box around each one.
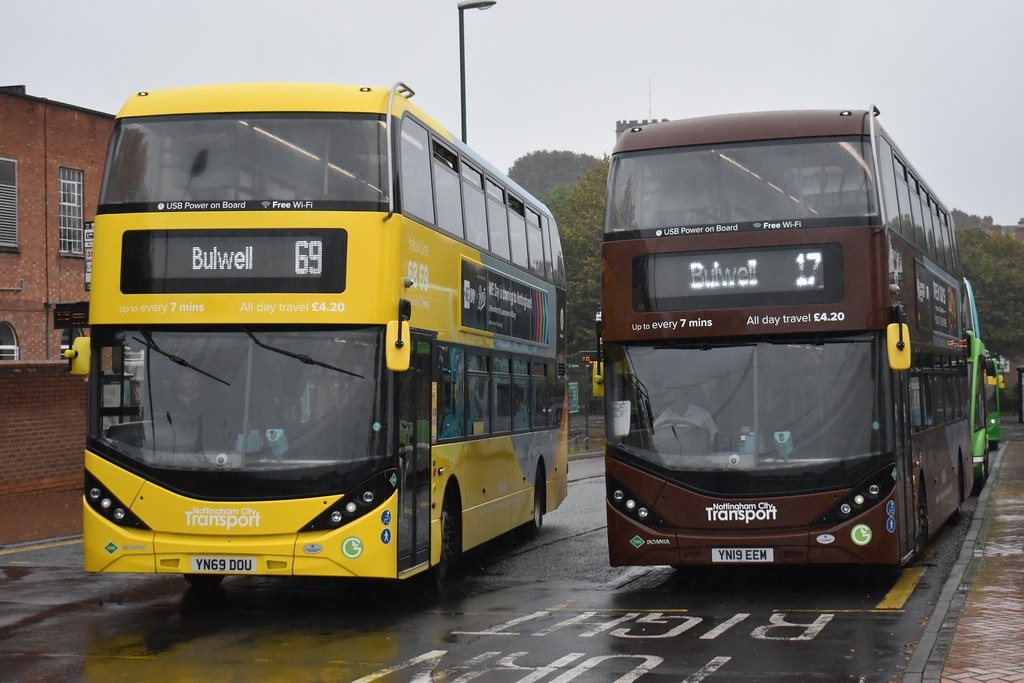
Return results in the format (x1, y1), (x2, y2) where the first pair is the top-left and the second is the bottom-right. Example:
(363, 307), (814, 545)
(456, 0), (499, 145)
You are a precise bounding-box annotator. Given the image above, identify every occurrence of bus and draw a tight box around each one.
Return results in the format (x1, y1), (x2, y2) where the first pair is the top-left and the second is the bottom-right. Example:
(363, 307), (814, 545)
(589, 102), (1010, 590)
(59, 79), (570, 600)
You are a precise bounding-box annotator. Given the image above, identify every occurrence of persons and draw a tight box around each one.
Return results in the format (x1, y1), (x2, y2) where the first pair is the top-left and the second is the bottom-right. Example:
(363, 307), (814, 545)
(653, 385), (720, 447)
